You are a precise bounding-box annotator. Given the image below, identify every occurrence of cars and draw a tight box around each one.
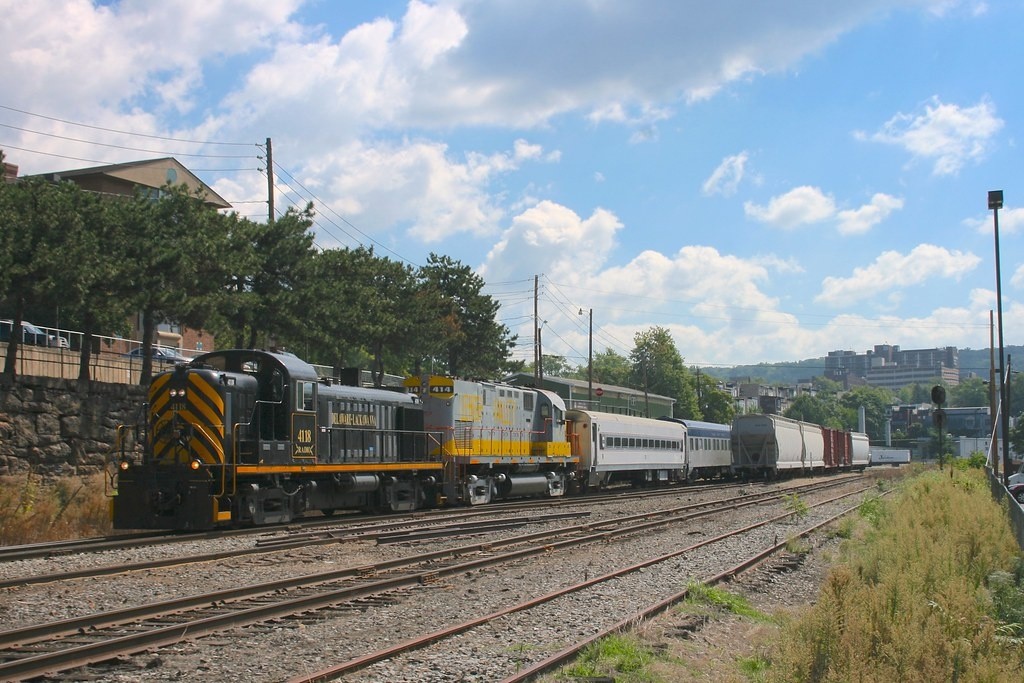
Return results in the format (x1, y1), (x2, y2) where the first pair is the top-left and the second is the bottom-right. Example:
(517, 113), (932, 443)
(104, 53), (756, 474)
(119, 346), (195, 366)
(1006, 462), (1024, 504)
(0, 318), (70, 350)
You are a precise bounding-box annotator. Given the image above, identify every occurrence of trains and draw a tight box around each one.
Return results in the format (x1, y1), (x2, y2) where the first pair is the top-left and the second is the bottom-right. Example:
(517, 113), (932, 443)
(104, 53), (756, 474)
(729, 412), (872, 483)
(103, 343), (732, 532)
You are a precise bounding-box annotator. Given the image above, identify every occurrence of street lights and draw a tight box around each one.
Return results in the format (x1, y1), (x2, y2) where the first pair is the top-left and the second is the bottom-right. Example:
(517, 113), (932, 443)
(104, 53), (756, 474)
(987, 189), (1009, 491)
(579, 308), (593, 412)
(538, 320), (548, 389)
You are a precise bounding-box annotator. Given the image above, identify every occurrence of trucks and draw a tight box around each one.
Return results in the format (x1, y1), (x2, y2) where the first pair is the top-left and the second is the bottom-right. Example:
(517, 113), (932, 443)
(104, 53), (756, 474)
(868, 449), (911, 467)
